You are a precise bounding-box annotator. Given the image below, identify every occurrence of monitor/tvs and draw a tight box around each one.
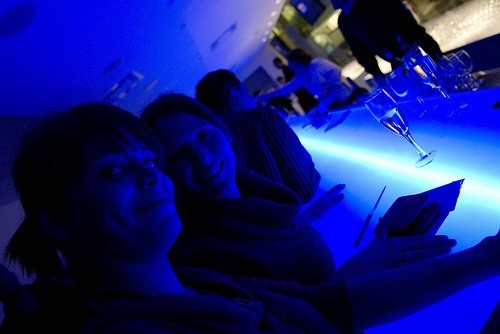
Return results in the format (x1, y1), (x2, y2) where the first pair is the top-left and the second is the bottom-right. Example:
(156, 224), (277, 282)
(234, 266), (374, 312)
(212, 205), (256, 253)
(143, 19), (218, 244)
(271, 37), (291, 58)
(289, 0), (326, 25)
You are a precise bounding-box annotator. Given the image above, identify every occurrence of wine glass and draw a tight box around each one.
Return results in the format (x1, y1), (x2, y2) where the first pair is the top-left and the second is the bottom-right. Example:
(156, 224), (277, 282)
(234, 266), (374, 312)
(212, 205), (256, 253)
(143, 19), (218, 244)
(364, 45), (482, 167)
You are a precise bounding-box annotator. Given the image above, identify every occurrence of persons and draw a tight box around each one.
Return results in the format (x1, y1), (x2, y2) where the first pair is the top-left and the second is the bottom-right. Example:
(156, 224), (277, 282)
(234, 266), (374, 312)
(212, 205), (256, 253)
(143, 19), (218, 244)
(143, 93), (458, 282)
(253, 0), (450, 114)
(0, 102), (500, 334)
(196, 69), (322, 205)
